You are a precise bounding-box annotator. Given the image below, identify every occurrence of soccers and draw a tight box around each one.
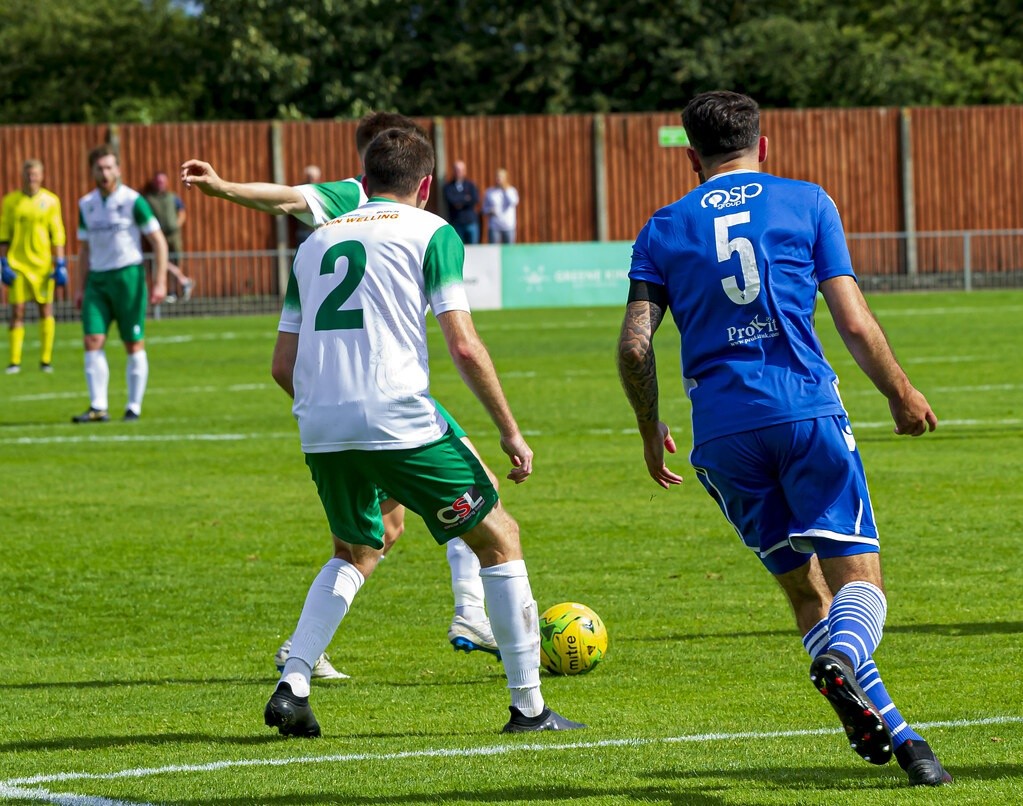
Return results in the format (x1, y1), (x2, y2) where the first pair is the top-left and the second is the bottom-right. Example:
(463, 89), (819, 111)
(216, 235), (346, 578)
(539, 602), (610, 676)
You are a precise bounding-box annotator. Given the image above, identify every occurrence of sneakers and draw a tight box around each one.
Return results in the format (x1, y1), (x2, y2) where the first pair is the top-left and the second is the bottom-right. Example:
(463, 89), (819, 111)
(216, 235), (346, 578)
(264, 681), (322, 738)
(73, 407), (110, 423)
(123, 409), (139, 420)
(501, 706), (587, 734)
(894, 739), (952, 787)
(275, 639), (351, 679)
(448, 615), (502, 663)
(809, 649), (894, 765)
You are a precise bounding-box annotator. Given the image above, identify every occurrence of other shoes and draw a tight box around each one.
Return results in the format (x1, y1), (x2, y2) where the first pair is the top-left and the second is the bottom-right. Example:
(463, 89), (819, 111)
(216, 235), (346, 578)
(164, 293), (178, 305)
(40, 362), (53, 373)
(5, 364), (21, 374)
(179, 279), (196, 304)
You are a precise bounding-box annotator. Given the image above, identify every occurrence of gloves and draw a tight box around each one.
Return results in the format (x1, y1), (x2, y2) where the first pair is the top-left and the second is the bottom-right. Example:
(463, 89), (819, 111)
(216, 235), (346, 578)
(50, 259), (69, 287)
(0, 257), (16, 285)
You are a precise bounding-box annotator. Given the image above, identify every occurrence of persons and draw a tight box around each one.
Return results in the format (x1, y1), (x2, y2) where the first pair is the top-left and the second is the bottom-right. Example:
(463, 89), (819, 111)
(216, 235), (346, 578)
(615, 92), (954, 786)
(72, 146), (168, 423)
(0, 159), (68, 375)
(142, 172), (194, 305)
(441, 158), (523, 244)
(263, 125), (590, 738)
(289, 165), (322, 252)
(177, 108), (502, 682)
